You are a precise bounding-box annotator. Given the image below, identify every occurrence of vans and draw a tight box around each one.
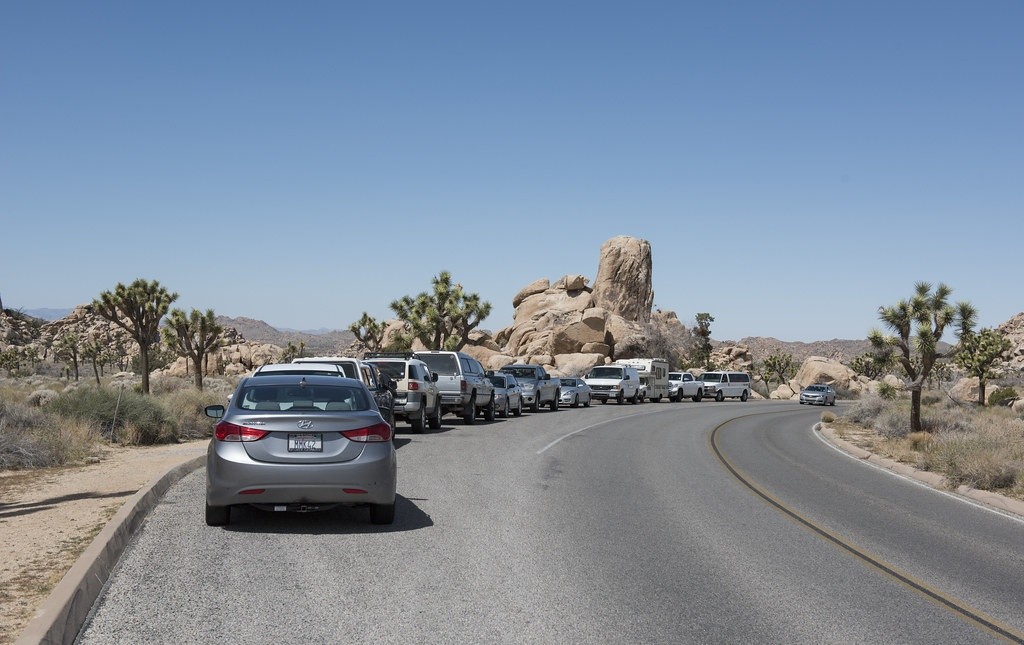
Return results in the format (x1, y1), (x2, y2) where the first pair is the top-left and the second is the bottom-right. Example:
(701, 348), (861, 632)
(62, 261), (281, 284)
(584, 364), (641, 405)
(695, 370), (751, 402)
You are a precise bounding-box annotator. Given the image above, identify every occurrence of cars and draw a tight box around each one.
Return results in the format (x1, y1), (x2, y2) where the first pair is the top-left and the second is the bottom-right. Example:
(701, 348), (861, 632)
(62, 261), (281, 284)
(556, 377), (593, 408)
(228, 356), (398, 442)
(203, 375), (398, 527)
(487, 373), (523, 418)
(799, 383), (836, 406)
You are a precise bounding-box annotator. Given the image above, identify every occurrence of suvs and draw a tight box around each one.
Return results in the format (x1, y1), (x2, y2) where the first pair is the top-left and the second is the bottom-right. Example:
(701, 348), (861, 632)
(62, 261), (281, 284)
(362, 351), (443, 433)
(408, 350), (496, 424)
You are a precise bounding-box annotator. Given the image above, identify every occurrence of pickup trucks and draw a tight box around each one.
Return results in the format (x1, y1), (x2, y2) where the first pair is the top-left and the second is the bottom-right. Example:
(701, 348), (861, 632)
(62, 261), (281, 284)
(669, 371), (705, 402)
(498, 364), (561, 412)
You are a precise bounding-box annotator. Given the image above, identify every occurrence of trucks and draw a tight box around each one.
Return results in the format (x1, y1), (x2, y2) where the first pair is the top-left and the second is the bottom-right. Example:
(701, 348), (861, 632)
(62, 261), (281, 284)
(616, 358), (669, 404)
